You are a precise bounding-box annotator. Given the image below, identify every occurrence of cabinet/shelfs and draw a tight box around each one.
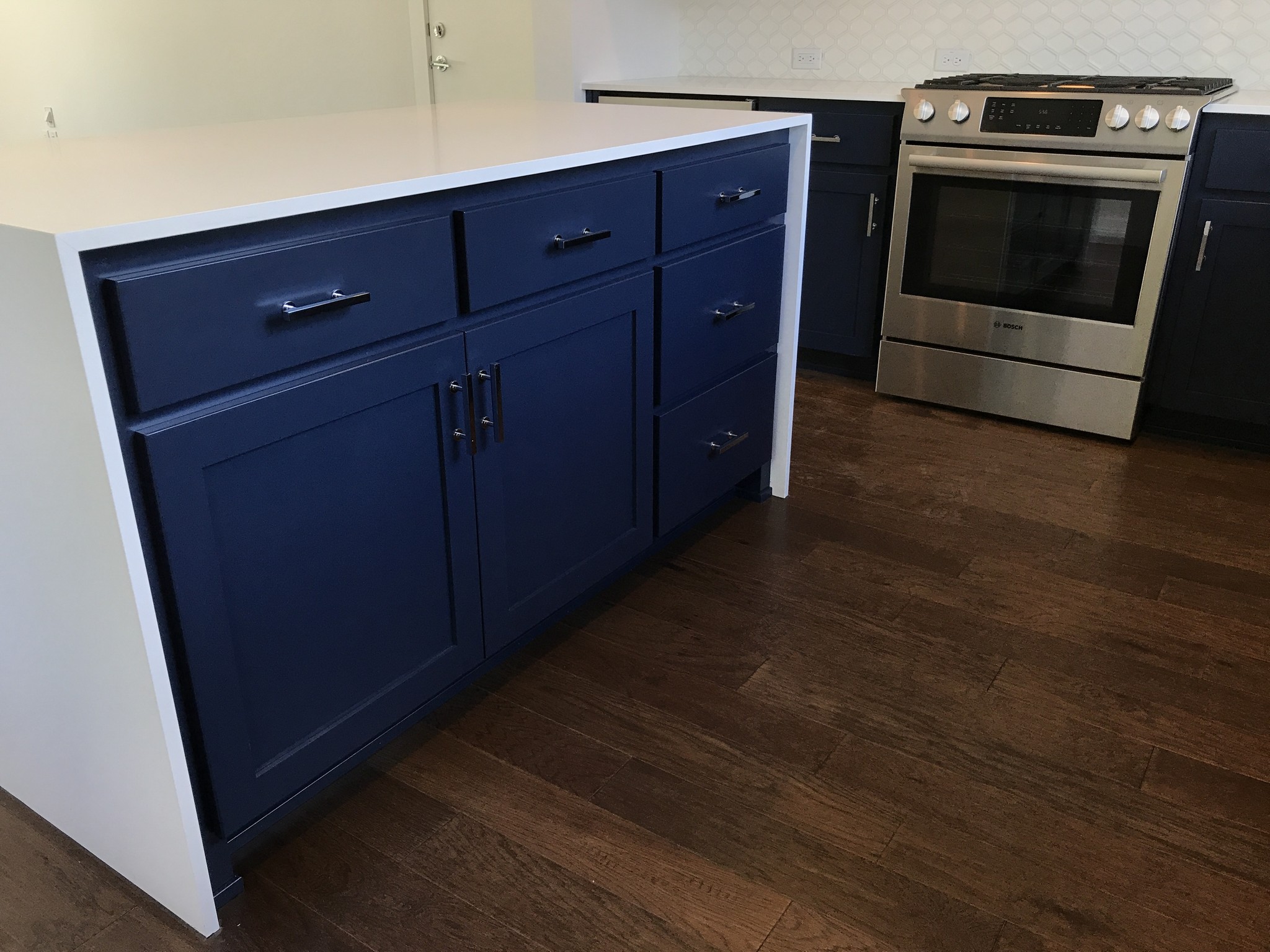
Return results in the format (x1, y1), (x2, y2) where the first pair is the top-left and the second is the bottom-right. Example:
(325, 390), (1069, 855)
(1139, 112), (1270, 447)
(758, 97), (905, 383)
(77, 127), (788, 911)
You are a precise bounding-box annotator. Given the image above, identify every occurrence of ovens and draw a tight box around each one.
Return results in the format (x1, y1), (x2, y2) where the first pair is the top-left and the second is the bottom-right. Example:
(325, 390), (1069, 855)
(875, 143), (1188, 443)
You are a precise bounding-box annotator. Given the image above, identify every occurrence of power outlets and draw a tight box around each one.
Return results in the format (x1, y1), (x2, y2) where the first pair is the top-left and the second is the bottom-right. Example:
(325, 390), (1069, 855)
(793, 48), (822, 69)
(934, 49), (969, 72)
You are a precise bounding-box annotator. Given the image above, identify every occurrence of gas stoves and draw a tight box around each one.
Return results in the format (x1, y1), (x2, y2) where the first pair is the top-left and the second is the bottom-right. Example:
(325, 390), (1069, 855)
(899, 73), (1246, 169)
